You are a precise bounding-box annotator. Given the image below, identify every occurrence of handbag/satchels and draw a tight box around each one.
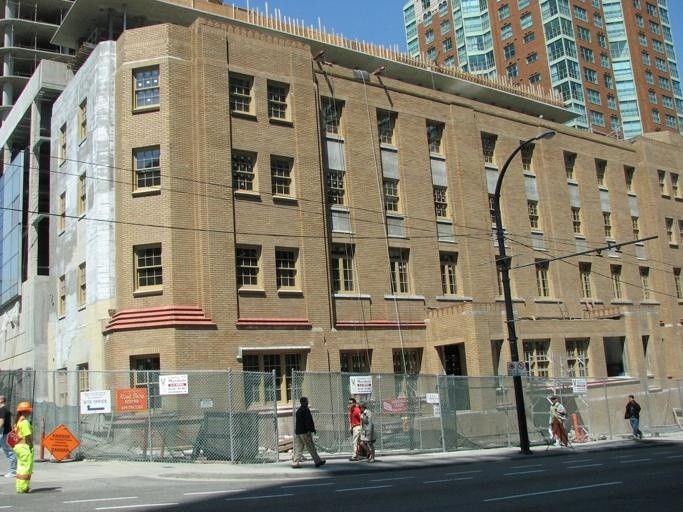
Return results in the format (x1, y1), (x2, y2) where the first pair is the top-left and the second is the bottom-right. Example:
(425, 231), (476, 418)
(555, 409), (566, 417)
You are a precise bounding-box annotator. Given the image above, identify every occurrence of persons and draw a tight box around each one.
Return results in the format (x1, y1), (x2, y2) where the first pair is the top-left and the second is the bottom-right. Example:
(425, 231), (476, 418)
(357, 400), (376, 463)
(549, 395), (567, 447)
(0, 394), (16, 478)
(624, 394), (642, 440)
(9, 402), (34, 494)
(347, 397), (362, 461)
(290, 396), (326, 468)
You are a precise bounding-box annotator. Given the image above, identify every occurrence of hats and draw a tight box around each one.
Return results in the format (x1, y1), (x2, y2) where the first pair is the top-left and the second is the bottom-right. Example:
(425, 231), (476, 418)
(550, 396), (557, 400)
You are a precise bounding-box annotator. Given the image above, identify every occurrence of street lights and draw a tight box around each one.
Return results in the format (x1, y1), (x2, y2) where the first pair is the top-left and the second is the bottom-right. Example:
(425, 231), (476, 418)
(494, 128), (557, 454)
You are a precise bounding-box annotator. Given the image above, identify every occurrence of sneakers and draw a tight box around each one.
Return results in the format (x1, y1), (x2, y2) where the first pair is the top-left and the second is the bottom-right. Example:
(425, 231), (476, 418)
(17, 488), (33, 494)
(553, 441), (566, 446)
(293, 460), (326, 468)
(634, 432), (642, 440)
(5, 473), (16, 477)
(349, 456), (375, 463)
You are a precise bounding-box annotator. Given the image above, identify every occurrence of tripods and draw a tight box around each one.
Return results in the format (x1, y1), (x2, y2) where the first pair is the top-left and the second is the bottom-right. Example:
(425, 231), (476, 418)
(544, 425), (575, 452)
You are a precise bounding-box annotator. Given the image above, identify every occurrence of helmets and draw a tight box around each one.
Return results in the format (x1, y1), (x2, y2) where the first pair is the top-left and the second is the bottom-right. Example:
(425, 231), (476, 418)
(17, 402), (32, 411)
